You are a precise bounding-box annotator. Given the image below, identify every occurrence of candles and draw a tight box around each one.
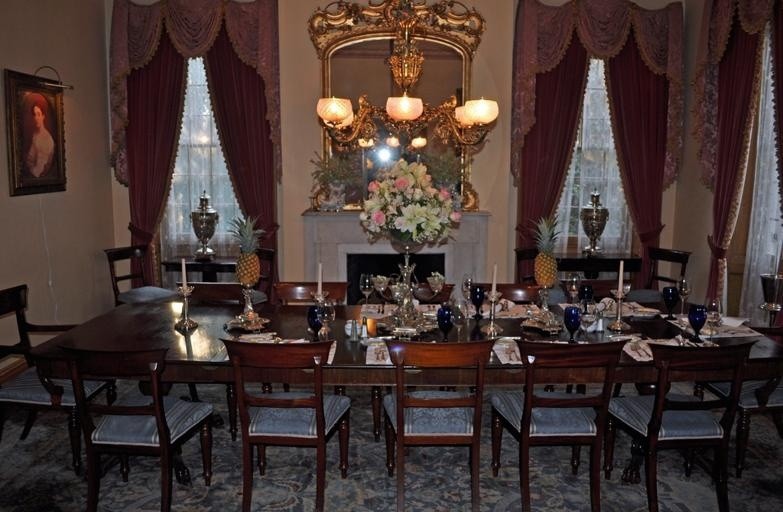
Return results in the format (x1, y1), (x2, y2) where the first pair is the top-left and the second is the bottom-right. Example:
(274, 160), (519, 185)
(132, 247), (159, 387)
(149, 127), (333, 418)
(316, 263), (324, 297)
(491, 263), (497, 294)
(181, 258), (187, 288)
(618, 260), (624, 292)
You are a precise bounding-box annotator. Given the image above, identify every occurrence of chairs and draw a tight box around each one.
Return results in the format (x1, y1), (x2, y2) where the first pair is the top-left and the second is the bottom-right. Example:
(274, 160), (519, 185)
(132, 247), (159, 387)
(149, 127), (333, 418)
(491, 339), (627, 512)
(175, 280), (249, 302)
(692, 322), (782, 478)
(470, 282), (545, 304)
(602, 340), (760, 512)
(58, 345), (213, 512)
(219, 337), (353, 512)
(383, 338), (494, 512)
(646, 247), (692, 294)
(559, 277), (630, 303)
(552, 250), (643, 278)
(1, 285), (116, 479)
(270, 282), (350, 304)
(162, 254), (238, 282)
(370, 283), (456, 304)
(104, 245), (177, 306)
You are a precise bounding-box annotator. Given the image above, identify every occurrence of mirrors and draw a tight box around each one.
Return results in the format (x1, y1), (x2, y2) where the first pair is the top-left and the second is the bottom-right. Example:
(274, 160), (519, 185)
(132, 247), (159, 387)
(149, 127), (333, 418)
(307, 0), (481, 212)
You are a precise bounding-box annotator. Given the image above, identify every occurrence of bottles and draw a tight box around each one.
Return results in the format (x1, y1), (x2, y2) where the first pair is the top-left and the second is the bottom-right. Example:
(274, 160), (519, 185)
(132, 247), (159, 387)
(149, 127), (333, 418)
(593, 309), (603, 331)
(350, 319), (358, 341)
(360, 317), (366, 337)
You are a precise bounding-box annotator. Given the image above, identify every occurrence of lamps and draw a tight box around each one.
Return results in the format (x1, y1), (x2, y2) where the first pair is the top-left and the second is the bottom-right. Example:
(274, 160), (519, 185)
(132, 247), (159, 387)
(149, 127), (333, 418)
(315, 1), (499, 152)
(189, 191), (220, 260)
(578, 188), (607, 252)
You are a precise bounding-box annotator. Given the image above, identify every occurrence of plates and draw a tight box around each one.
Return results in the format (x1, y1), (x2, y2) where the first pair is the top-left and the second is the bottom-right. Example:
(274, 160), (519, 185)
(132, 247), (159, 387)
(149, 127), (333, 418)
(631, 307), (662, 317)
(230, 317), (270, 329)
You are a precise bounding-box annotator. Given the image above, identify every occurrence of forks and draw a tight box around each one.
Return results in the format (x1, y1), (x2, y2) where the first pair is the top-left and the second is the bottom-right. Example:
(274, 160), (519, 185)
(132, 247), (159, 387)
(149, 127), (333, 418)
(630, 341), (651, 358)
(505, 348), (520, 362)
(374, 345), (386, 360)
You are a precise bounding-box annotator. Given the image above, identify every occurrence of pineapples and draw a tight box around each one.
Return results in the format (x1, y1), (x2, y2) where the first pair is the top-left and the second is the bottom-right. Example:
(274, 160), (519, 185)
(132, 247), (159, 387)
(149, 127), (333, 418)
(224, 214), (264, 288)
(525, 214), (565, 288)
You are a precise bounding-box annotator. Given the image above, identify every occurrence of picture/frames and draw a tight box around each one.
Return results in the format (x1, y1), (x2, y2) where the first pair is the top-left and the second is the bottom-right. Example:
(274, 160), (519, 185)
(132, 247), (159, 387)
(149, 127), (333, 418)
(5, 65), (74, 196)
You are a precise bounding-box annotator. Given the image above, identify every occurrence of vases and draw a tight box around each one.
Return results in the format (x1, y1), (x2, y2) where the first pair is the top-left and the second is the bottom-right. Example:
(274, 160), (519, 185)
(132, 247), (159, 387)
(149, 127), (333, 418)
(391, 239), (425, 255)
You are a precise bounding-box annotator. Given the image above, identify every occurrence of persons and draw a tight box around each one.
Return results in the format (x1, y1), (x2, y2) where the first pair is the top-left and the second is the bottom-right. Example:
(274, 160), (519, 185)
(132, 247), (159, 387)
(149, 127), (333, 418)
(25, 102), (54, 178)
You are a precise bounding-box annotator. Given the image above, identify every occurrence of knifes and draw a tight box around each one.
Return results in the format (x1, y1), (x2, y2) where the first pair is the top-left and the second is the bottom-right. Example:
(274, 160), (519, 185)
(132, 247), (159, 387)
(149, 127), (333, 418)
(674, 338), (685, 347)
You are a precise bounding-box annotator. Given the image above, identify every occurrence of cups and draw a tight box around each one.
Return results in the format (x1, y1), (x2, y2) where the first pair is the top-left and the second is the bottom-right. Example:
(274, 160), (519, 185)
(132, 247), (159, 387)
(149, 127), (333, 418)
(251, 318), (260, 333)
(344, 319), (362, 337)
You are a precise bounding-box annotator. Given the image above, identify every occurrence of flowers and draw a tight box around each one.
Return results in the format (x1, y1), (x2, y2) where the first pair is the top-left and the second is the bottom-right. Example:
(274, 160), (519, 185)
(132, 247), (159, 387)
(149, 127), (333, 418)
(359, 155), (460, 249)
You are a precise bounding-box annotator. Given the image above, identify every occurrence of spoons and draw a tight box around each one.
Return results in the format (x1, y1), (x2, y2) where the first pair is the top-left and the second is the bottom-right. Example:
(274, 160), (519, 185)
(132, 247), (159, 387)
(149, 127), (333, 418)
(522, 305), (532, 315)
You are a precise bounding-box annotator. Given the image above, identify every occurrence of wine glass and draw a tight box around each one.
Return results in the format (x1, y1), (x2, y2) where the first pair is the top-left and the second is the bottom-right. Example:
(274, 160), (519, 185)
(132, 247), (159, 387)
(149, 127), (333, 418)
(469, 317), (483, 342)
(306, 297), (335, 342)
(688, 304), (705, 342)
(663, 287), (676, 320)
(707, 298), (719, 344)
(359, 272), (374, 315)
(461, 274), (484, 319)
(450, 299), (466, 343)
(564, 306), (580, 343)
(567, 273), (579, 304)
(676, 276), (690, 318)
(578, 299), (596, 341)
(437, 307), (453, 341)
(579, 284), (592, 315)
